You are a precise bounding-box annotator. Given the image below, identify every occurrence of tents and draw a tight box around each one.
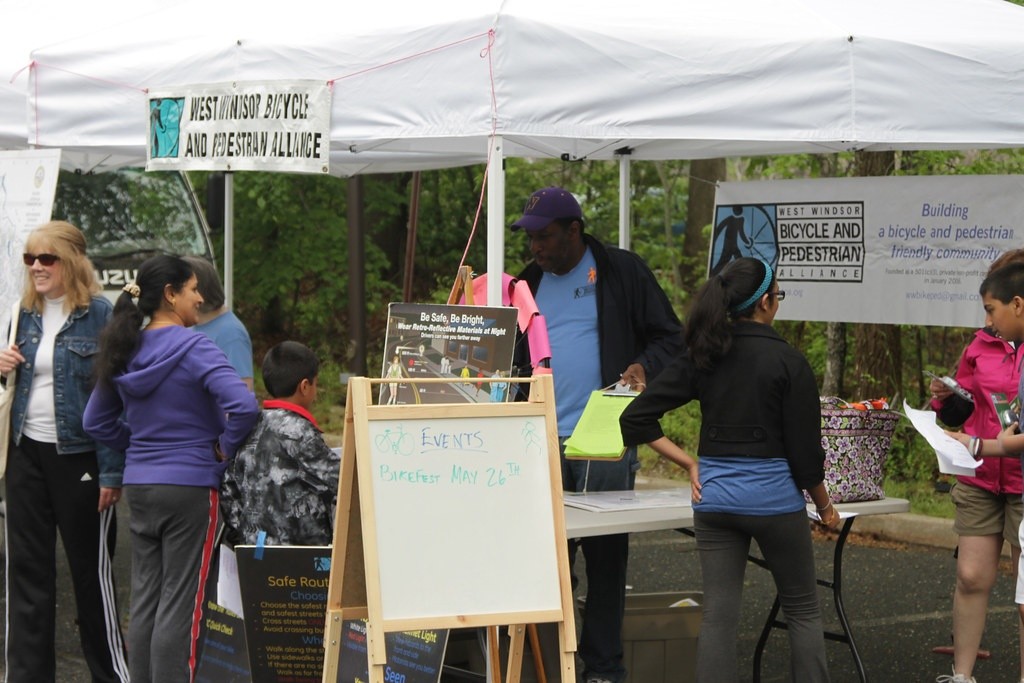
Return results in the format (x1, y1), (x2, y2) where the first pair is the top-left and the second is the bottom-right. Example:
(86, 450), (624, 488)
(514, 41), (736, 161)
(1, 0), (1024, 314)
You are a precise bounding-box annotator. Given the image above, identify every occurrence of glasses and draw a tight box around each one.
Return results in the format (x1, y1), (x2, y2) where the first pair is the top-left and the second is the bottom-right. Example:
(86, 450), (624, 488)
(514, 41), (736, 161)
(24, 253), (63, 267)
(757, 290), (786, 305)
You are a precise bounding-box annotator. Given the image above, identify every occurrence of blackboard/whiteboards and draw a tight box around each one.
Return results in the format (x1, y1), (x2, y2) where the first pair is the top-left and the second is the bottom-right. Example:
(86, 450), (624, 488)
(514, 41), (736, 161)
(326, 402), (574, 632)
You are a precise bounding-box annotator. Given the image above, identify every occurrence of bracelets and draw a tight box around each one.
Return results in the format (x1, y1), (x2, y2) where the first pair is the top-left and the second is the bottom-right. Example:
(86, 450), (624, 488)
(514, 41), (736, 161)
(818, 499), (831, 511)
(969, 436), (983, 460)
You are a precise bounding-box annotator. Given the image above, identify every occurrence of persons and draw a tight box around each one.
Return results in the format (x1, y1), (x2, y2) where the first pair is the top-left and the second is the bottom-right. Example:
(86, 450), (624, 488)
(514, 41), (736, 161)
(83, 256), (261, 683)
(0, 219), (130, 683)
(513, 188), (686, 683)
(222, 340), (341, 544)
(931, 249), (1024, 683)
(620, 257), (840, 683)
(178, 256), (254, 394)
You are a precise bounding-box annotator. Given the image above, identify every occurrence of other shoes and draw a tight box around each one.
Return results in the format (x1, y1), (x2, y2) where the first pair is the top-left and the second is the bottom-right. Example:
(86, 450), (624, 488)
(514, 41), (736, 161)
(936, 664), (976, 683)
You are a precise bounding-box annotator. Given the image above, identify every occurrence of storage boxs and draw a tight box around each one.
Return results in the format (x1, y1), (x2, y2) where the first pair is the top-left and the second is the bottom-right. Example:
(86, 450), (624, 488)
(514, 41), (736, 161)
(577, 589), (704, 683)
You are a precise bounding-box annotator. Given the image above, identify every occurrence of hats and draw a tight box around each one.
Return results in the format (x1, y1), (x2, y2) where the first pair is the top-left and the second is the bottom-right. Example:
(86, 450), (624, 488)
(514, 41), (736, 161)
(510, 186), (583, 233)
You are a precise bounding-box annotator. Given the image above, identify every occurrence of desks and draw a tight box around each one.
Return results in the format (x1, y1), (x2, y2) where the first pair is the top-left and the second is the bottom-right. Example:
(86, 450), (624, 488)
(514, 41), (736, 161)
(443, 486), (910, 683)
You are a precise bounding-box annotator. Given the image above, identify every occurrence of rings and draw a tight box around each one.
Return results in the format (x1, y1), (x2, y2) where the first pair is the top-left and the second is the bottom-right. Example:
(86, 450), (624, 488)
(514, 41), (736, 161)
(113, 497), (116, 500)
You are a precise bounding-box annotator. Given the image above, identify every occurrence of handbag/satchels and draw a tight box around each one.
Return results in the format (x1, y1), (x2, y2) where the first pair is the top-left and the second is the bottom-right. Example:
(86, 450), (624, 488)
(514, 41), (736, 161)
(801, 395), (901, 504)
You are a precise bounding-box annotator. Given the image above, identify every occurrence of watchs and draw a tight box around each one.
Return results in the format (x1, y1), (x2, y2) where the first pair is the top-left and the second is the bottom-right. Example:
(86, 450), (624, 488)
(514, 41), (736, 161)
(815, 501), (835, 526)
